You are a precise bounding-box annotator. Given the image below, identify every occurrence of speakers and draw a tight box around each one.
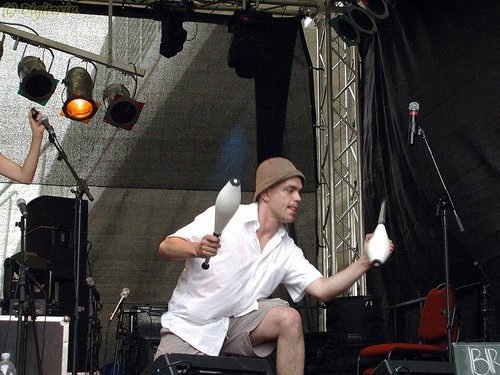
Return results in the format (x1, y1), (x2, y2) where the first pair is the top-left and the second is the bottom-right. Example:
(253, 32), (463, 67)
(370, 359), (456, 374)
(138, 353), (274, 375)
(21, 195), (88, 279)
(11, 275), (90, 372)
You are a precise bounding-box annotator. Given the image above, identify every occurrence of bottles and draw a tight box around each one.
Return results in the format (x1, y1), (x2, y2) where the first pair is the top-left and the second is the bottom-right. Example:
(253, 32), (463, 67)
(0, 353), (17, 375)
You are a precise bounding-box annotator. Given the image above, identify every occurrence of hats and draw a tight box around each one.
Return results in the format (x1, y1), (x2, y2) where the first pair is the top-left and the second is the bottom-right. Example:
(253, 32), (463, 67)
(254, 158), (306, 201)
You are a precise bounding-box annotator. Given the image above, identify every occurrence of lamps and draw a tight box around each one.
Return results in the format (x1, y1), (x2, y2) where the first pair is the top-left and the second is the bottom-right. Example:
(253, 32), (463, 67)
(16, 42), (60, 106)
(224, 7), (273, 76)
(102, 68), (147, 132)
(60, 55), (103, 125)
(348, 5), (379, 36)
(362, 0), (392, 20)
(328, 13), (362, 49)
(158, 10), (187, 59)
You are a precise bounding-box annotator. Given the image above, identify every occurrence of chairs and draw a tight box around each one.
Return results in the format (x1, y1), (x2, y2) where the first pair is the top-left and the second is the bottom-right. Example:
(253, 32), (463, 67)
(355, 282), (465, 375)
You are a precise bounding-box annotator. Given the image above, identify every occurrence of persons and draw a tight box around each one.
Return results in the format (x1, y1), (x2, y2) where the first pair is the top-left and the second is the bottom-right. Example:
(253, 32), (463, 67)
(153, 156), (394, 375)
(0, 111), (45, 185)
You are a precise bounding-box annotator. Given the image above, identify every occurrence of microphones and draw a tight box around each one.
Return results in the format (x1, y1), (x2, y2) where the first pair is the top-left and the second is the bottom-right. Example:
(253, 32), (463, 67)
(107, 287), (131, 322)
(407, 100), (420, 145)
(17, 198), (28, 218)
(87, 275), (100, 302)
(38, 115), (58, 139)
